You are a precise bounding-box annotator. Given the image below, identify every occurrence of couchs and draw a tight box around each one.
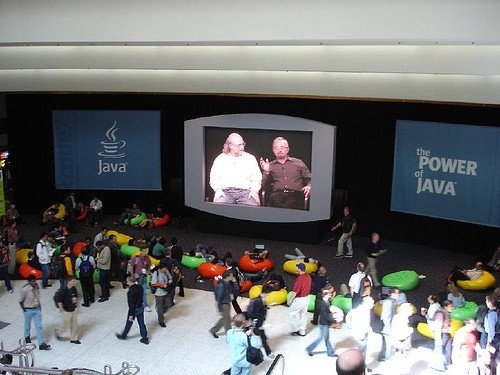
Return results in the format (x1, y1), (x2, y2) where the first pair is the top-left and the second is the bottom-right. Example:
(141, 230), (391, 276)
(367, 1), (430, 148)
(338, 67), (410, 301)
(381, 270), (419, 291)
(149, 214), (168, 228)
(74, 205), (87, 222)
(73, 230), (162, 282)
(374, 300), (417, 319)
(180, 255), (217, 270)
(283, 259), (318, 275)
(457, 270), (496, 290)
(43, 204), (65, 220)
(417, 319), (464, 340)
(331, 294), (353, 314)
(250, 284), (287, 306)
(197, 262), (229, 279)
(449, 301), (479, 324)
(287, 292), (317, 311)
(213, 276), (252, 293)
(238, 255), (273, 272)
(123, 211), (147, 224)
(15, 248), (50, 279)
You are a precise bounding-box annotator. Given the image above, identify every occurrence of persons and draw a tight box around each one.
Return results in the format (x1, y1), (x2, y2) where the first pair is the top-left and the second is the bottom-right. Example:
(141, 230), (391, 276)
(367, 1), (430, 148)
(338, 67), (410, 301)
(36, 192), (121, 307)
(19, 274), (50, 350)
(55, 274), (81, 344)
(364, 232), (382, 287)
(210, 248), (312, 375)
(116, 275), (148, 344)
(305, 262), (415, 374)
(209, 132), (263, 206)
(332, 207), (357, 258)
(118, 203), (164, 229)
(421, 247), (500, 375)
(260, 137), (311, 210)
(0, 204), (19, 293)
(127, 234), (212, 327)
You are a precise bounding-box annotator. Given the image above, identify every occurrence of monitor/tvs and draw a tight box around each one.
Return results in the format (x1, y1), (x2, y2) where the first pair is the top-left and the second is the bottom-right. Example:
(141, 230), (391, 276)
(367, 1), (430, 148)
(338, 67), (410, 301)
(184, 112), (337, 224)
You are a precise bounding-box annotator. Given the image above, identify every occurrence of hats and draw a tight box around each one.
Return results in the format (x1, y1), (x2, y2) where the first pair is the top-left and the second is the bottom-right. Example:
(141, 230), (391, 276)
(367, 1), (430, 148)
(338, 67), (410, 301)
(296, 263), (306, 271)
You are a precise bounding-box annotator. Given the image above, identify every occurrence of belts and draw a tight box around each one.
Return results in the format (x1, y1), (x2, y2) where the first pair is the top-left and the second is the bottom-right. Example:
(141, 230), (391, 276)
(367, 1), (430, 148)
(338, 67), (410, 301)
(275, 189), (297, 193)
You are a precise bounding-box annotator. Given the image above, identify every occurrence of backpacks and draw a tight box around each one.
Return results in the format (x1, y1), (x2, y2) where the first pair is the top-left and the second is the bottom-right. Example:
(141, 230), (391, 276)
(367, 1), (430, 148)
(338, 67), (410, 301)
(27, 241), (44, 271)
(79, 256), (94, 279)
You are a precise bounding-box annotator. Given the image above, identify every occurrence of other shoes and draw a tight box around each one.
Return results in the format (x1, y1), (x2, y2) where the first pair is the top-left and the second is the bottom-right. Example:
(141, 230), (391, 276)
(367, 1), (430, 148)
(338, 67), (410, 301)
(39, 342), (51, 350)
(144, 306), (151, 312)
(25, 336), (31, 343)
(70, 339), (81, 344)
(160, 322), (166, 327)
(81, 298), (95, 307)
(98, 296), (109, 302)
(140, 339), (149, 344)
(116, 334), (124, 339)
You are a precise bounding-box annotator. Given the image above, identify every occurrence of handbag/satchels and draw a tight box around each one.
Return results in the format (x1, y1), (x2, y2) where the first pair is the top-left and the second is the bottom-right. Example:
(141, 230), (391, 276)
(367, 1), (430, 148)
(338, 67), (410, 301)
(246, 345), (264, 366)
(149, 270), (159, 294)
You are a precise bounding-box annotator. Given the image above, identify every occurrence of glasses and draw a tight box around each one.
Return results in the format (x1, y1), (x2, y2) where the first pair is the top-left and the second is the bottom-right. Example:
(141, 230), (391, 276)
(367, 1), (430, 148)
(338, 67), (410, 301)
(232, 142), (247, 147)
(273, 146), (288, 150)
